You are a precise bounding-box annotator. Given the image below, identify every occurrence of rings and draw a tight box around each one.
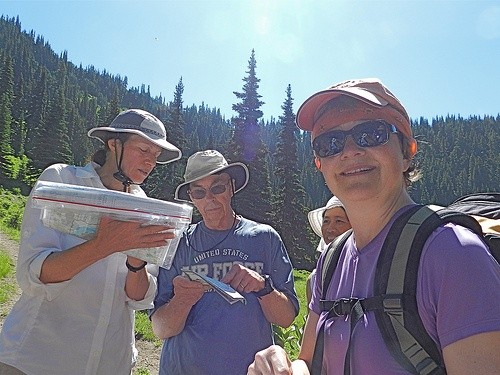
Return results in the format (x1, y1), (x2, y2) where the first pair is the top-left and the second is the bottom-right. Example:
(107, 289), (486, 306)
(239, 283), (245, 289)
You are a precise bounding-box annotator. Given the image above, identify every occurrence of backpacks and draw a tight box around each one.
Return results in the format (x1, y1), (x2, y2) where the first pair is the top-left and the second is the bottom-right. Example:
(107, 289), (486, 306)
(319, 193), (500, 375)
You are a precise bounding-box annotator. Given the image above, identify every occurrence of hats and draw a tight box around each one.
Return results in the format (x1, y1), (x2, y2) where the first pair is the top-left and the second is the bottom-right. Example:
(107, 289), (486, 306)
(88, 109), (183, 164)
(307, 195), (343, 239)
(296, 78), (412, 146)
(175, 150), (249, 203)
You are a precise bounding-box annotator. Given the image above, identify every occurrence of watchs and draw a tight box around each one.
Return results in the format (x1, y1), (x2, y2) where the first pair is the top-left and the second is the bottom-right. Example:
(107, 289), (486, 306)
(253, 274), (274, 300)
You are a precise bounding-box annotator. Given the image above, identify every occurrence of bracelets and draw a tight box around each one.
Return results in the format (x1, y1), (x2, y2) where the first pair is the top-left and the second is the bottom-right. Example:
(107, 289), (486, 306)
(125, 260), (147, 272)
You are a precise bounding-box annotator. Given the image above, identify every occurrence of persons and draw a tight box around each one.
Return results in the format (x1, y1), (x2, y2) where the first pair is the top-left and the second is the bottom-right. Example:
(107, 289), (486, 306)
(247, 77), (500, 375)
(148, 149), (300, 375)
(306, 196), (352, 314)
(331, 138), (339, 153)
(0, 109), (182, 375)
(360, 133), (369, 146)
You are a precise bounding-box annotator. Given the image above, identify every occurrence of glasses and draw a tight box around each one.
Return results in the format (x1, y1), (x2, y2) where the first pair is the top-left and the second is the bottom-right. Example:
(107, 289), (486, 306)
(311, 119), (403, 158)
(188, 179), (231, 199)
